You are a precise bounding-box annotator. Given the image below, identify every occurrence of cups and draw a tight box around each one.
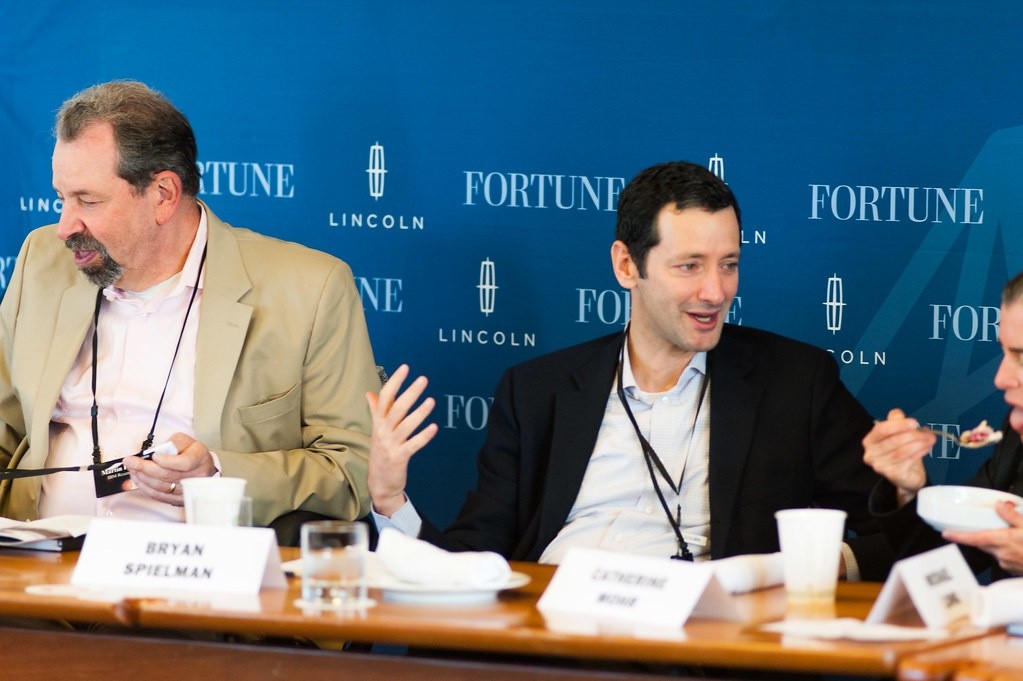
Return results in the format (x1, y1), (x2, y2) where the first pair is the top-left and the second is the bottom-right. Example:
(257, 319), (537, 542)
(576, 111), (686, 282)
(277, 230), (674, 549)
(180, 477), (247, 526)
(774, 507), (847, 602)
(302, 522), (370, 617)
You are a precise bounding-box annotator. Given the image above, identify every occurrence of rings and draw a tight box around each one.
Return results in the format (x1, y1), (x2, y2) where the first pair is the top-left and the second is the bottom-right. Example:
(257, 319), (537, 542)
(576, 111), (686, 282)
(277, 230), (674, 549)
(169, 482), (176, 493)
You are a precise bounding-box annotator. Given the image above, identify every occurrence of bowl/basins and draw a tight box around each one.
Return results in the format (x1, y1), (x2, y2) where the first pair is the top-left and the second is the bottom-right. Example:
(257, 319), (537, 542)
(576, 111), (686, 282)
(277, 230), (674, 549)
(917, 486), (1023, 534)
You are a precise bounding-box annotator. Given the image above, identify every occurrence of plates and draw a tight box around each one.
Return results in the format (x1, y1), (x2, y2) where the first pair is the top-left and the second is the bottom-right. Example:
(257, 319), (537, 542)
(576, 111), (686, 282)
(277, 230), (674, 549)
(373, 572), (532, 603)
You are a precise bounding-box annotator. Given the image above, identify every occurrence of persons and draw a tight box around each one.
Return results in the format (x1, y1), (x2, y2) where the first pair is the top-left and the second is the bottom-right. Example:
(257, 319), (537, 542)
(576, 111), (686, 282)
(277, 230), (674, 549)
(367, 160), (885, 583)
(863, 273), (1023, 583)
(0, 82), (382, 548)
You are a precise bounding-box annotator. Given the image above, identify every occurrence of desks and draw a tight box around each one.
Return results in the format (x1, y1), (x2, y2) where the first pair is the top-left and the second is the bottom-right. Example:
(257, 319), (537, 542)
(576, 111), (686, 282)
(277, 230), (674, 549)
(0, 536), (1021, 681)
